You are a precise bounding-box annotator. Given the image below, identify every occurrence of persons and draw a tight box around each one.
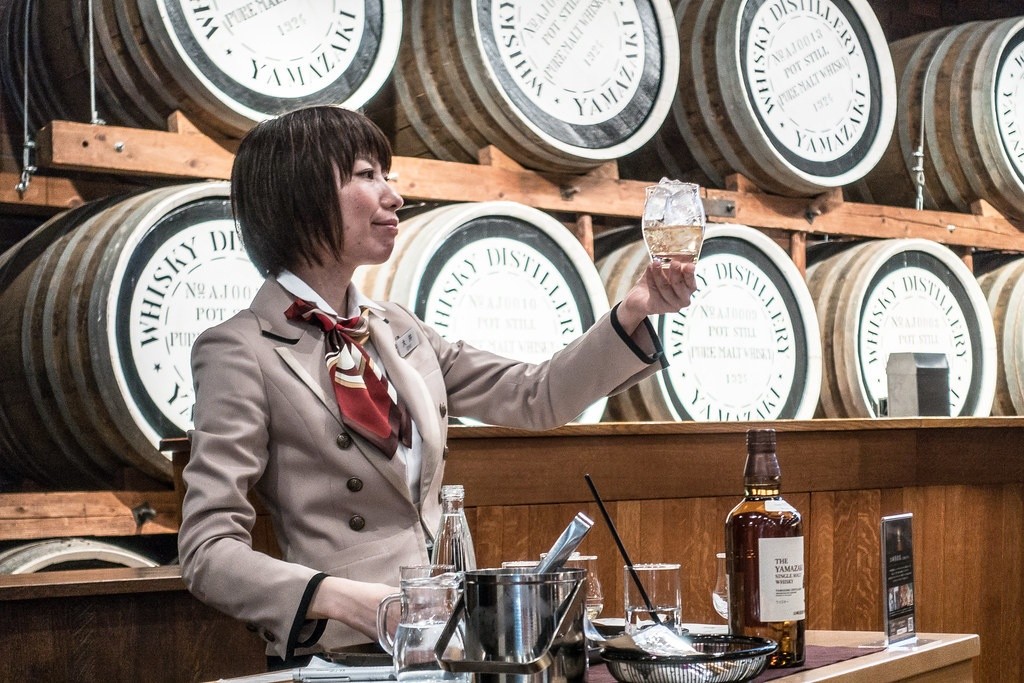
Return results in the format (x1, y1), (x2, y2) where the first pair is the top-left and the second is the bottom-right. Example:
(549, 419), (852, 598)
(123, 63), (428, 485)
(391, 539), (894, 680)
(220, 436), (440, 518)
(176, 105), (698, 675)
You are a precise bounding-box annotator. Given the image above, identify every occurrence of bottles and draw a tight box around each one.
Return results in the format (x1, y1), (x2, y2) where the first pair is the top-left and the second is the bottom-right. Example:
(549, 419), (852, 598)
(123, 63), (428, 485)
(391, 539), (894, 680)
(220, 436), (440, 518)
(427, 483), (475, 573)
(376, 564), (473, 683)
(725, 427), (808, 669)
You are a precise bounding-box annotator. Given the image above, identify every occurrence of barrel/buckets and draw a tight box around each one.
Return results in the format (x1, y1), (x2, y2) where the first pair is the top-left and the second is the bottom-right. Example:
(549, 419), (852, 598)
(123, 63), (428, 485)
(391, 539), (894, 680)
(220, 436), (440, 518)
(977, 254), (1024, 417)
(350, 201), (613, 426)
(2, 526), (166, 574)
(364, 0), (680, 173)
(618, 0), (897, 196)
(807, 237), (1000, 419)
(434, 564), (589, 683)
(843, 17), (1024, 222)
(0, 180), (265, 489)
(591, 222), (821, 420)
(0, 0), (403, 144)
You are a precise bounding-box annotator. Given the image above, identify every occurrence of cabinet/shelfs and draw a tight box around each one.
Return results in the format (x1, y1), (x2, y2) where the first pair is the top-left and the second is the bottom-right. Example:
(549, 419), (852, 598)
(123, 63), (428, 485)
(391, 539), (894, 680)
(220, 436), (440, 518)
(0, 104), (1024, 683)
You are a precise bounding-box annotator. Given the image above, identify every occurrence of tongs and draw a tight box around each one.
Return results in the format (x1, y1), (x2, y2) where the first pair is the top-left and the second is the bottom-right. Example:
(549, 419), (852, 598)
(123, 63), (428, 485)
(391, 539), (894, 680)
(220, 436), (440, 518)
(531, 513), (595, 575)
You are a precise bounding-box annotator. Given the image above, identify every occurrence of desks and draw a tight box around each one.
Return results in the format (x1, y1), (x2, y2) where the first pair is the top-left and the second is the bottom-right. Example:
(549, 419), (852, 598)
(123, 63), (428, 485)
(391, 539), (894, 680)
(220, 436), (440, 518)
(194, 624), (981, 683)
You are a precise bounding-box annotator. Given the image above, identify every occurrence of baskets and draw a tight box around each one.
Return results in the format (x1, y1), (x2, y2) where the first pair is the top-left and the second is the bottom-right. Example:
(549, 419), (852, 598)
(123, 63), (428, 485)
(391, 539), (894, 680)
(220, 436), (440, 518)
(600, 633), (778, 683)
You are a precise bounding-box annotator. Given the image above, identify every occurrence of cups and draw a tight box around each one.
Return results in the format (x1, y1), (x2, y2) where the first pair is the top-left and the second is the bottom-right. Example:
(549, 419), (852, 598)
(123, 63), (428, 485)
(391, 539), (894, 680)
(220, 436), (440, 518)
(539, 551), (602, 627)
(641, 182), (707, 276)
(621, 563), (684, 638)
(500, 557), (539, 571)
(711, 552), (731, 623)
